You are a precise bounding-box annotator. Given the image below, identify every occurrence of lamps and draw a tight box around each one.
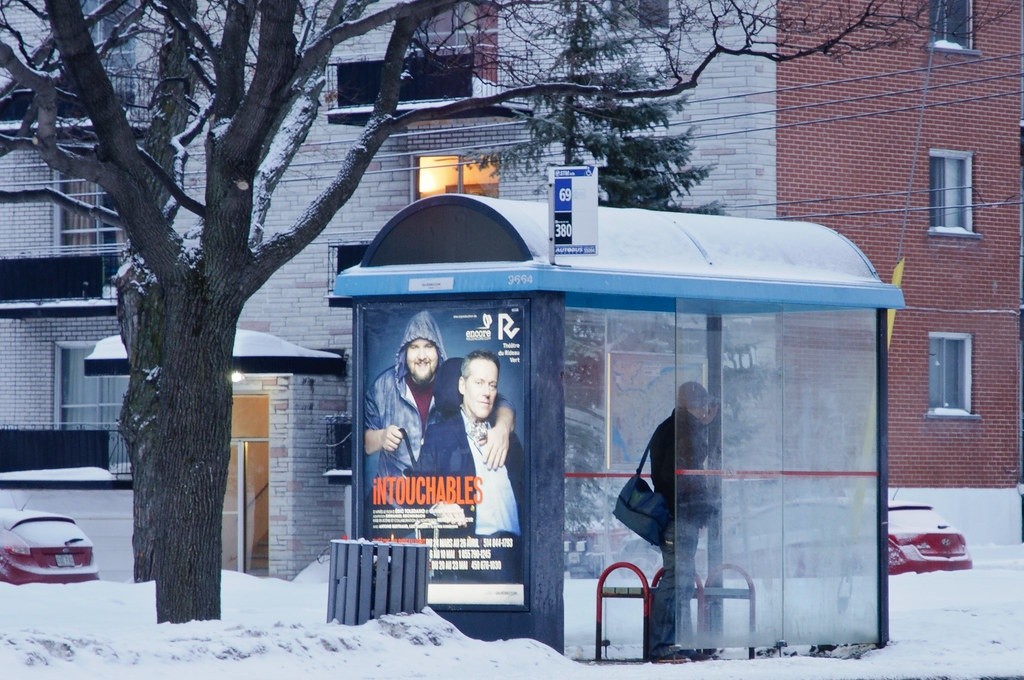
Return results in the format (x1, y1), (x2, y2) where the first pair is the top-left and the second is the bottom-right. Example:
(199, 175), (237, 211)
(930, 354), (941, 367)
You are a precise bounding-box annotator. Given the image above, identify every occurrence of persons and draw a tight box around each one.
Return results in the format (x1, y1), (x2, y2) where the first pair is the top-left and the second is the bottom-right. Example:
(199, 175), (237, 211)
(651, 380), (719, 664)
(363, 310), (524, 539)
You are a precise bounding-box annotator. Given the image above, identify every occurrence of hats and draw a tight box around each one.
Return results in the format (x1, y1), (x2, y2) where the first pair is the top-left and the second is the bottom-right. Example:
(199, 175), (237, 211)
(678, 381), (709, 408)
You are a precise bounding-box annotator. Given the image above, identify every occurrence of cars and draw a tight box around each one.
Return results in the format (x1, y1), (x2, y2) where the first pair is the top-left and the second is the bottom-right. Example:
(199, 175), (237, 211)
(0, 505), (101, 586)
(719, 494), (974, 582)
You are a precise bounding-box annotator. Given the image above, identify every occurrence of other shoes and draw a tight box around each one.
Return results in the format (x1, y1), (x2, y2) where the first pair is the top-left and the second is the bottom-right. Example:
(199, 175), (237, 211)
(655, 652), (693, 663)
(679, 649), (713, 662)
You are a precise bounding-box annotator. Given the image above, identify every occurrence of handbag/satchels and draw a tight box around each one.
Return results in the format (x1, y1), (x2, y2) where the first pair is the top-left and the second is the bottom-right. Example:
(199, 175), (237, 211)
(613, 471), (675, 546)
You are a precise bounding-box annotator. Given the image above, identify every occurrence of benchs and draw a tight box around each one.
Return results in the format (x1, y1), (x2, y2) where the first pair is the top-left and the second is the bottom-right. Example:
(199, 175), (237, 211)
(595, 561), (756, 660)
